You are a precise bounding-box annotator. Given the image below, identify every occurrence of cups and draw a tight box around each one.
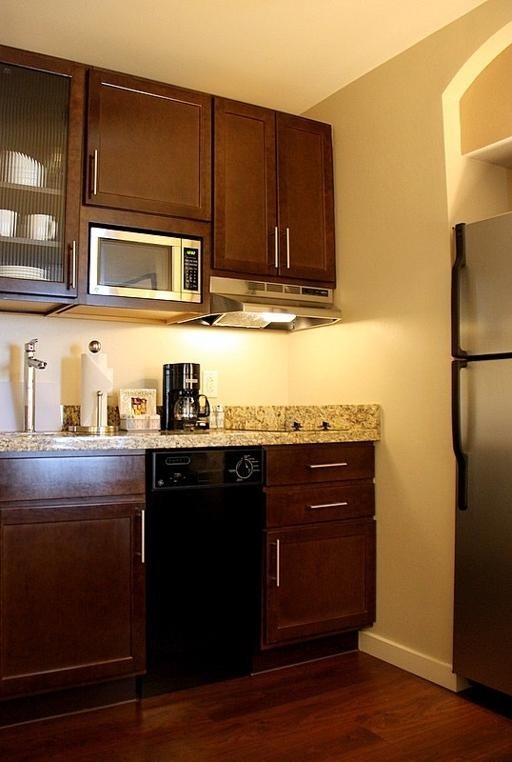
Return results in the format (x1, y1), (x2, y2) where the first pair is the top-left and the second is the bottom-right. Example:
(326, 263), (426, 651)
(1, 209), (18, 239)
(24, 214), (57, 241)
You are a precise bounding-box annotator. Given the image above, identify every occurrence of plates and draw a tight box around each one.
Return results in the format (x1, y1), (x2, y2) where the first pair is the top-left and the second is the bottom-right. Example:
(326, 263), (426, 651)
(0, 264), (52, 281)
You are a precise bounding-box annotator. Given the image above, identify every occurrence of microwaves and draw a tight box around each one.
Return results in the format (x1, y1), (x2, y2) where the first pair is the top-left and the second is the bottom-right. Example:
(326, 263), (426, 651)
(89, 226), (203, 304)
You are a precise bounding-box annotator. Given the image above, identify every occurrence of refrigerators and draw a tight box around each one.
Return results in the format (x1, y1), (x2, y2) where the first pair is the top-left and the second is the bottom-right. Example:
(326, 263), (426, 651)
(450, 211), (512, 711)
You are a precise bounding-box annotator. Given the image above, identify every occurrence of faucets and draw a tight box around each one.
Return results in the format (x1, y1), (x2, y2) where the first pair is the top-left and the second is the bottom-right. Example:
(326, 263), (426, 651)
(23, 338), (46, 432)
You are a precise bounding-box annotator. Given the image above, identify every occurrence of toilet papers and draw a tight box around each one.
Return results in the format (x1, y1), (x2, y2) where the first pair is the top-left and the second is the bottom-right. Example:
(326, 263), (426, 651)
(80, 351), (114, 428)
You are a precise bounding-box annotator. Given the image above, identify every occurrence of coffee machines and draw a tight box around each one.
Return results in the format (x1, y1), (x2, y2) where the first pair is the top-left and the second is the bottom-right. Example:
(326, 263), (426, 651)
(162, 363), (210, 431)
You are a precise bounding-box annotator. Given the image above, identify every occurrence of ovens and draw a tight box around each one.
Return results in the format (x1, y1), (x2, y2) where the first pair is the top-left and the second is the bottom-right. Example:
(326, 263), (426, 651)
(145, 447), (265, 695)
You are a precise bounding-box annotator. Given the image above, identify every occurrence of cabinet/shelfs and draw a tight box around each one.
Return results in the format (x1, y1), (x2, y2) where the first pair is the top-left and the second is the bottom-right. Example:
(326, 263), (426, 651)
(267, 448), (382, 643)
(0, 44), (76, 303)
(209, 97), (334, 286)
(0, 507), (145, 692)
(85, 70), (207, 222)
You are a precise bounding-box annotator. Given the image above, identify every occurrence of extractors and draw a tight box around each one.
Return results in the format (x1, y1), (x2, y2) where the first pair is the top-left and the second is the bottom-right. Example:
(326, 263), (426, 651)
(177, 276), (344, 334)
